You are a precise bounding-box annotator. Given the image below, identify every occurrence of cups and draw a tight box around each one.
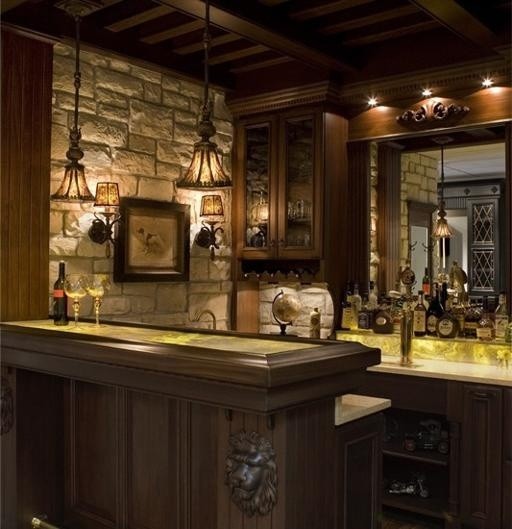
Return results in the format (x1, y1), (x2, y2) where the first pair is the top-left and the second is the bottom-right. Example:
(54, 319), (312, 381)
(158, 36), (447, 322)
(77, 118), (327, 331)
(63, 273), (88, 330)
(86, 272), (111, 329)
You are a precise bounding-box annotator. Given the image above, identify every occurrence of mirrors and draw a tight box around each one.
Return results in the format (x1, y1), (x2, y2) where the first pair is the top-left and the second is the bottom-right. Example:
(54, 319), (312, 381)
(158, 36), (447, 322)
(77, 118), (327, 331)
(367, 135), (512, 303)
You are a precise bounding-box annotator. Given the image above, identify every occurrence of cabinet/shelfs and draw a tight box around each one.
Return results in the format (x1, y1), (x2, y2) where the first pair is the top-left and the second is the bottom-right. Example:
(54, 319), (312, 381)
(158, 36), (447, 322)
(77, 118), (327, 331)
(367, 358), (512, 529)
(232, 78), (349, 260)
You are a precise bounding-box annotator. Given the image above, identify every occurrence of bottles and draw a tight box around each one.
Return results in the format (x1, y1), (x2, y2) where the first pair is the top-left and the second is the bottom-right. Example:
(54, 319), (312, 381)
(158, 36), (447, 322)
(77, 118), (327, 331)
(414, 291), (426, 336)
(309, 307), (321, 341)
(453, 299), (486, 338)
(341, 287), (353, 331)
(368, 280), (378, 314)
(53, 263), (68, 325)
(426, 281), (443, 336)
(422, 268), (431, 298)
(358, 299), (372, 332)
(349, 286), (362, 333)
(373, 299), (393, 334)
(478, 296), (495, 341)
(495, 293), (509, 341)
(437, 300), (459, 339)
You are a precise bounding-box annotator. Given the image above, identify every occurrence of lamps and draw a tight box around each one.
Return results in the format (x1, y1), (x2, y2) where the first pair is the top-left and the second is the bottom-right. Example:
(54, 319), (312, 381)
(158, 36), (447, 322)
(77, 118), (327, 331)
(194, 193), (227, 262)
(433, 199), (453, 237)
(51, 162), (97, 203)
(88, 182), (122, 257)
(177, 140), (232, 192)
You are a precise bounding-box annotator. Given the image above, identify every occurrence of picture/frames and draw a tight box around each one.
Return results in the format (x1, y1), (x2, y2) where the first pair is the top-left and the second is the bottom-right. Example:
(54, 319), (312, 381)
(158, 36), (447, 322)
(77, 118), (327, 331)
(112, 195), (191, 283)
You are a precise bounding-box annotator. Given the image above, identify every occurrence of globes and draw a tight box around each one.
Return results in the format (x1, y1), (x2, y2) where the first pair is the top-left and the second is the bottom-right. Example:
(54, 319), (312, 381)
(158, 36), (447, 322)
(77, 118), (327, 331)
(271, 290), (303, 339)
